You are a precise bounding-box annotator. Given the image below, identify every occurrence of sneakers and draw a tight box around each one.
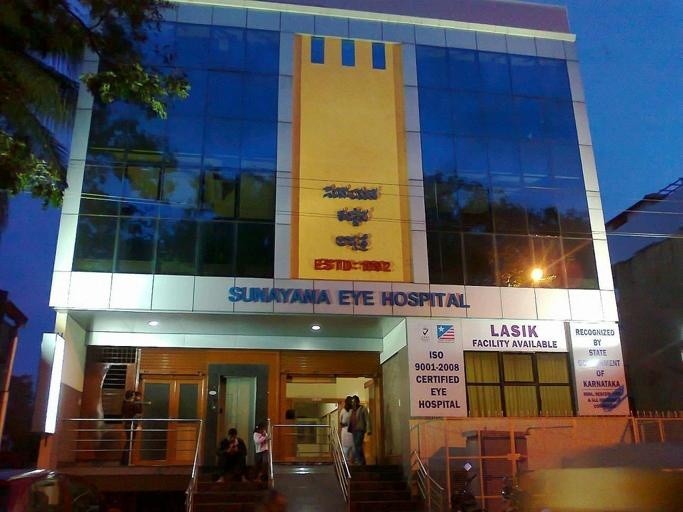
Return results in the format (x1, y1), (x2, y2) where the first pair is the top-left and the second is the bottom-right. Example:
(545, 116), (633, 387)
(215, 477), (225, 481)
(241, 476), (248, 482)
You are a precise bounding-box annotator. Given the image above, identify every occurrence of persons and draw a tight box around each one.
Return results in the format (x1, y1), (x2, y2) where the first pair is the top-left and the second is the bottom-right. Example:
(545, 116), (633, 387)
(216, 428), (248, 483)
(340, 396), (371, 465)
(121, 389), (144, 465)
(253, 423), (270, 481)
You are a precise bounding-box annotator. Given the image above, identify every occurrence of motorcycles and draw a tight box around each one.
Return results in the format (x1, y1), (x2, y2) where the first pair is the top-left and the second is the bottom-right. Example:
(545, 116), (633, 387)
(448, 473), (488, 512)
(498, 469), (549, 512)
